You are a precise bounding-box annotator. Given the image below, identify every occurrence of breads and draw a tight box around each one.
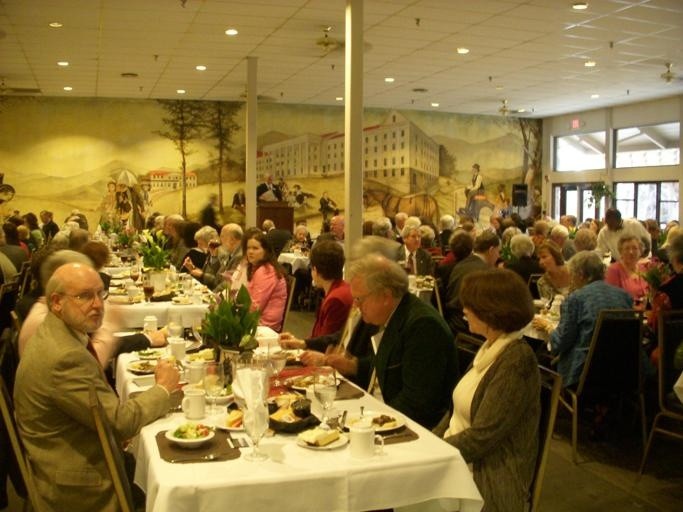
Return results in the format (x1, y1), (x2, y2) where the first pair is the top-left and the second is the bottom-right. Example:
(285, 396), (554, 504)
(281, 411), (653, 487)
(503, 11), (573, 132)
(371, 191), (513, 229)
(305, 429), (339, 448)
(269, 394), (303, 423)
(227, 408), (244, 425)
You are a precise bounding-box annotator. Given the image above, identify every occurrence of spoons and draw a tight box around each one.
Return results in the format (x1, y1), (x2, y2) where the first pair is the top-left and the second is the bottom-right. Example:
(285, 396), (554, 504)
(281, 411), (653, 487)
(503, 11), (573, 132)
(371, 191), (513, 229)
(169, 451), (225, 463)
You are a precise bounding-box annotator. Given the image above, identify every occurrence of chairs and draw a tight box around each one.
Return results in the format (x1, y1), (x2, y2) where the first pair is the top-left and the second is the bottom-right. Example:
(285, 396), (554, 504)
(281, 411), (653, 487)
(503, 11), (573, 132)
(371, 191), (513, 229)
(530, 361), (564, 511)
(556, 306), (651, 465)
(635, 303), (683, 479)
(87, 382), (140, 512)
(0, 386), (44, 512)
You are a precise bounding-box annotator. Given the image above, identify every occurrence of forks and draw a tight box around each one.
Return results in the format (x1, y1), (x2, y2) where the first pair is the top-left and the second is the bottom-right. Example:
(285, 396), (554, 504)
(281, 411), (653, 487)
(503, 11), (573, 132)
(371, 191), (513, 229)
(359, 405), (365, 418)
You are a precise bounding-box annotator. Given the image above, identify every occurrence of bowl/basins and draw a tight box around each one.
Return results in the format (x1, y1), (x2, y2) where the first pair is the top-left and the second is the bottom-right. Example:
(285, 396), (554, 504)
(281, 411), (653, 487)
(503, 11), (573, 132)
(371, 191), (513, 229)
(164, 424), (215, 449)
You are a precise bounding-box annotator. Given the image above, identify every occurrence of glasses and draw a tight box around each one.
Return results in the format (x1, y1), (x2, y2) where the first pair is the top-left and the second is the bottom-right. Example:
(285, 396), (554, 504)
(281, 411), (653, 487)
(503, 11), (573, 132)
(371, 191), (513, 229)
(352, 291), (372, 307)
(58, 290), (109, 303)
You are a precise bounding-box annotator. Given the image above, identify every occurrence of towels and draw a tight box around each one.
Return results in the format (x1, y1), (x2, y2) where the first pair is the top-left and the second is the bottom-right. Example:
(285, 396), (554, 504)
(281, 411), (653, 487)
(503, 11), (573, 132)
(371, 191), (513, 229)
(229, 367), (268, 411)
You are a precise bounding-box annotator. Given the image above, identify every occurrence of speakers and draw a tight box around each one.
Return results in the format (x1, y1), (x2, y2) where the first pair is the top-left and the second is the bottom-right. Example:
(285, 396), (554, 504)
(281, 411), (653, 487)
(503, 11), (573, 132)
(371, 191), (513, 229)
(512, 184), (528, 206)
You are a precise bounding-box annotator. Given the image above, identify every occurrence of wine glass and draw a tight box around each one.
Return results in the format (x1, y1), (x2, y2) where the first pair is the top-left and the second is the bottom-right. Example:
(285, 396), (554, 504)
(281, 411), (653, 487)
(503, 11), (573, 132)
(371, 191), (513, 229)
(170, 269), (192, 297)
(265, 340), (288, 382)
(112, 238), (154, 304)
(313, 369), (337, 419)
(202, 361), (226, 415)
(237, 370), (272, 465)
(167, 314), (183, 334)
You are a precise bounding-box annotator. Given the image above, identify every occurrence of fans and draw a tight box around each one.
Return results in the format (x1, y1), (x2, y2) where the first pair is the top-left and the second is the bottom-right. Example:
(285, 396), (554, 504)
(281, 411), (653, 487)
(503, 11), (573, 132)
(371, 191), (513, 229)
(486, 98), (535, 116)
(647, 62), (683, 87)
(294, 23), (371, 60)
(0, 76), (45, 97)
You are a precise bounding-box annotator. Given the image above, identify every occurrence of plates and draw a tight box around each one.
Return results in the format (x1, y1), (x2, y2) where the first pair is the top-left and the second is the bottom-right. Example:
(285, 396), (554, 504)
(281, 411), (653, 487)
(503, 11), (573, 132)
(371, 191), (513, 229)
(193, 278), (221, 304)
(212, 419), (246, 432)
(283, 374), (343, 392)
(125, 349), (164, 373)
(296, 428), (347, 450)
(339, 412), (406, 432)
(106, 257), (138, 304)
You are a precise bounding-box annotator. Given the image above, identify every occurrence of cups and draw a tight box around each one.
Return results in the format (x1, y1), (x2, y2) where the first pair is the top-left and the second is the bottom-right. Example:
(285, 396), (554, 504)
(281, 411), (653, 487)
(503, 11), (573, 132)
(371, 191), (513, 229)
(184, 361), (207, 387)
(122, 277), (139, 304)
(407, 275), (416, 287)
(180, 387), (207, 420)
(346, 422), (383, 459)
(141, 314), (160, 331)
(166, 336), (186, 359)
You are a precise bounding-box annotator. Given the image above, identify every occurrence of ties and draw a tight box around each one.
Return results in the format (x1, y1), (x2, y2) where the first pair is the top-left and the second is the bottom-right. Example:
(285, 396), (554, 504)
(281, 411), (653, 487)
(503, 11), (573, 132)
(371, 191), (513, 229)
(86, 341), (102, 366)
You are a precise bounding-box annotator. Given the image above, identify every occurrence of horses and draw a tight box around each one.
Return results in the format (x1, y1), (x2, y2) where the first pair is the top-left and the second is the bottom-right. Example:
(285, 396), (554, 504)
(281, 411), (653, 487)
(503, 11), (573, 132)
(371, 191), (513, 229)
(363, 186), (441, 228)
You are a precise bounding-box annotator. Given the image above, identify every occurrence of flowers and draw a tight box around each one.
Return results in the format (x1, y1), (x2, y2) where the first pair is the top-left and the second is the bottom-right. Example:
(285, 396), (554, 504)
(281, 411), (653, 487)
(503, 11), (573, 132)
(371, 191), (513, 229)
(634, 254), (675, 297)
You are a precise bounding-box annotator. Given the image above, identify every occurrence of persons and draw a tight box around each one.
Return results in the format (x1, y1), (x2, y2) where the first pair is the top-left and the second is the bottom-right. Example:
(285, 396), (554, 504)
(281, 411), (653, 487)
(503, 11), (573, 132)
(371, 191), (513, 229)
(325, 253), (461, 432)
(1, 160), (682, 373)
(545, 252), (657, 447)
(7, 262), (182, 511)
(432, 265), (543, 510)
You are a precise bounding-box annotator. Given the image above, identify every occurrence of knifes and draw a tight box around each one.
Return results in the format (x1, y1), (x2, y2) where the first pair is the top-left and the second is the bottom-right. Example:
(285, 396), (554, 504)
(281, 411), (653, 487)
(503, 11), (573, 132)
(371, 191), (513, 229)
(339, 409), (346, 429)
